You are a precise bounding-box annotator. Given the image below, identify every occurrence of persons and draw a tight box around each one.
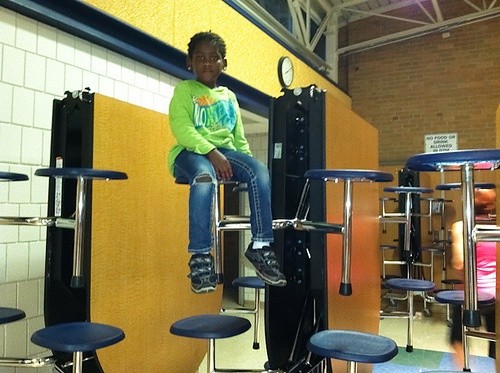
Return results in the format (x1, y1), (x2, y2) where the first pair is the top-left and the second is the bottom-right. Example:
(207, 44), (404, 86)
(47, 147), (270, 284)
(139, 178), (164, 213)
(168, 30), (287, 294)
(450, 189), (496, 369)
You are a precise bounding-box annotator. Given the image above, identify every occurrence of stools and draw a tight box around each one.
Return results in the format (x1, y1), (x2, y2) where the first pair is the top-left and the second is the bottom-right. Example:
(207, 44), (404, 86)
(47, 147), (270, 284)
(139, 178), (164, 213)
(0, 147), (500, 373)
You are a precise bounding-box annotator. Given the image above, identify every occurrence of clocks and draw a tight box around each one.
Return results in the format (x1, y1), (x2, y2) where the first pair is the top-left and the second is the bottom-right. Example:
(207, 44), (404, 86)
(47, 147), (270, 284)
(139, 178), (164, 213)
(278, 56), (294, 87)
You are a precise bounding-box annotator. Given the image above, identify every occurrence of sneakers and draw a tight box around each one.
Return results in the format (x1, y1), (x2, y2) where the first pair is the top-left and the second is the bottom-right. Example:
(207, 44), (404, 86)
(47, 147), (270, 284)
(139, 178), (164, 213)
(243, 241), (287, 286)
(188, 253), (218, 293)
